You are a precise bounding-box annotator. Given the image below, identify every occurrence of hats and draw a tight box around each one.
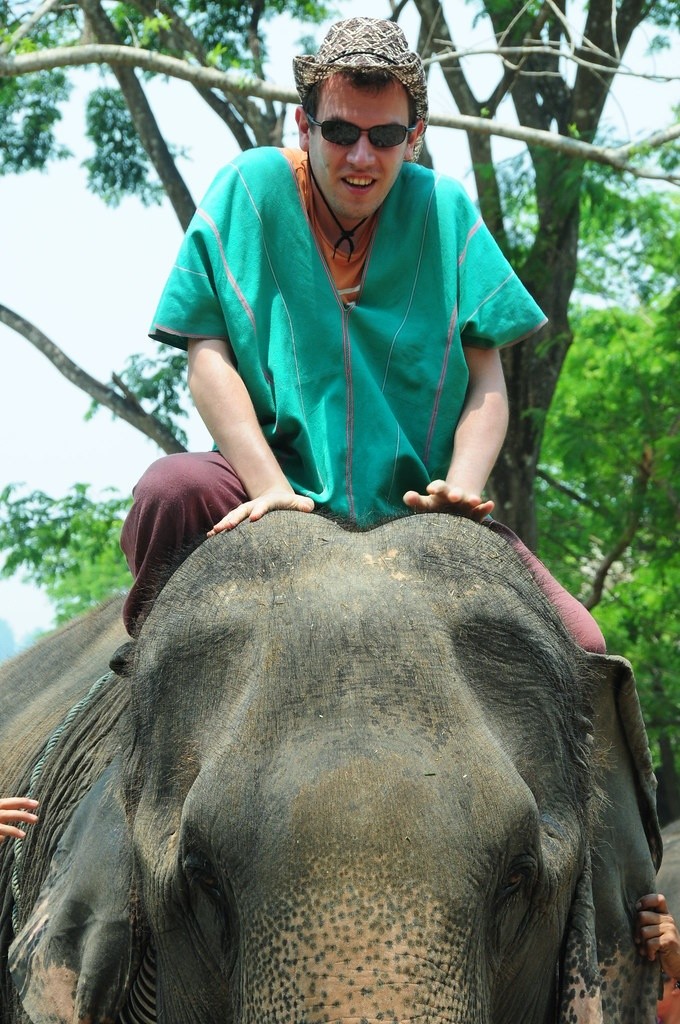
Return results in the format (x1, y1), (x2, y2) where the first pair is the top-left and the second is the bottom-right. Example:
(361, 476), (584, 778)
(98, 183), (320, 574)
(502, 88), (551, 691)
(292, 17), (429, 135)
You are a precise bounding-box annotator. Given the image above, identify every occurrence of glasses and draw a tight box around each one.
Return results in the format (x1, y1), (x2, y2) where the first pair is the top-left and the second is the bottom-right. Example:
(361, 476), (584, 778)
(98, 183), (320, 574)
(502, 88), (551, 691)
(306, 112), (416, 149)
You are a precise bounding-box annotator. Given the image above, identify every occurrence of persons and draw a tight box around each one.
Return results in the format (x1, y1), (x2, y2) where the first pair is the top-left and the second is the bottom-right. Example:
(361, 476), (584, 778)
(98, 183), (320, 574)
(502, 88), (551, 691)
(633, 893), (680, 1024)
(109, 17), (607, 676)
(0, 798), (40, 845)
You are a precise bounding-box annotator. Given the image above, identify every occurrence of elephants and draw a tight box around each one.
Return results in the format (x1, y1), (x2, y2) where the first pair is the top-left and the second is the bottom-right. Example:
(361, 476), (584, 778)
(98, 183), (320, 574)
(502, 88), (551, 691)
(0, 505), (663, 1024)
